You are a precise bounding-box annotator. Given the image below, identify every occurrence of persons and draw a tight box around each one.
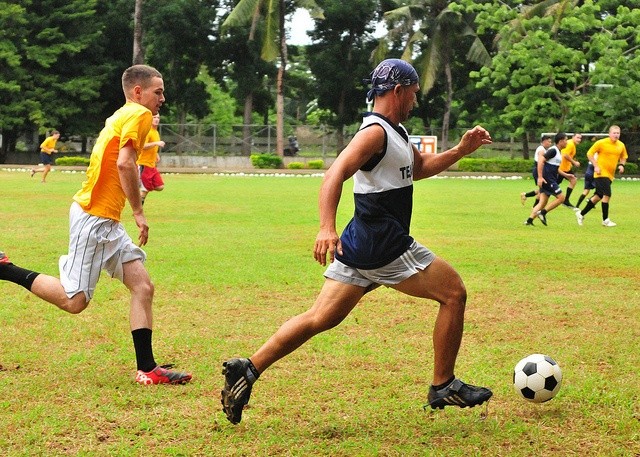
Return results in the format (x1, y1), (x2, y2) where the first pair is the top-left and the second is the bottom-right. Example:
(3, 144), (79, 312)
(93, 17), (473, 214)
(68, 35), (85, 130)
(28, 130), (60, 181)
(524, 132), (568, 226)
(521, 134), (552, 209)
(575, 148), (602, 207)
(219, 57), (493, 424)
(574, 123), (628, 226)
(140, 111), (164, 208)
(551, 132), (582, 209)
(1, 63), (193, 386)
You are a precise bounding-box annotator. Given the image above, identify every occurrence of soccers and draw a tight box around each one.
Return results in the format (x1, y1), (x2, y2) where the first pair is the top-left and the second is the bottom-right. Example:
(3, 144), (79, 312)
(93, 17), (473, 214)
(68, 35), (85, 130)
(513, 353), (562, 404)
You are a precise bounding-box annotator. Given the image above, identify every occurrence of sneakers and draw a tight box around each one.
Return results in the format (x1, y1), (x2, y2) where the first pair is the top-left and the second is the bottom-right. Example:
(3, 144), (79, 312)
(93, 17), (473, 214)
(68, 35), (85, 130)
(586, 198), (595, 208)
(601, 218), (618, 226)
(562, 201), (575, 208)
(573, 206), (580, 211)
(537, 211), (548, 226)
(521, 192), (526, 205)
(136, 366), (193, 386)
(428, 380), (493, 409)
(221, 357), (252, 426)
(525, 221), (534, 226)
(31, 170), (35, 177)
(0, 252), (11, 265)
(576, 210), (584, 225)
